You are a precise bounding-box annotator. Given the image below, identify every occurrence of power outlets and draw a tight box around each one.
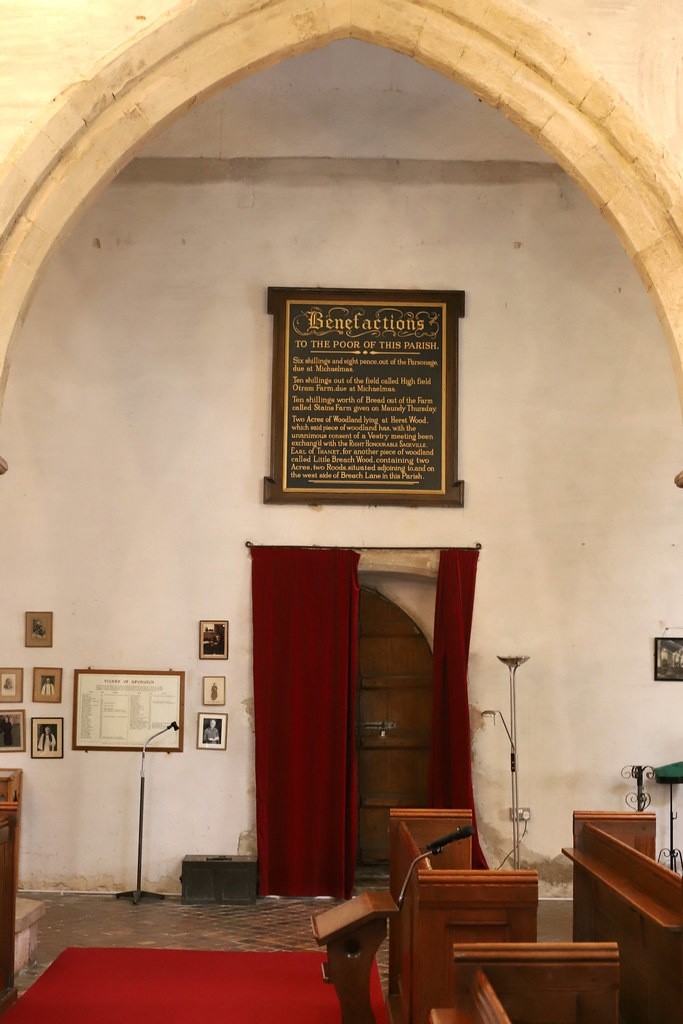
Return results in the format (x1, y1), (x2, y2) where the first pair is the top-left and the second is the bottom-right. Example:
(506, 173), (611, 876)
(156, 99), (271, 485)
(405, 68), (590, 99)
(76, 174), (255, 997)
(510, 807), (530, 820)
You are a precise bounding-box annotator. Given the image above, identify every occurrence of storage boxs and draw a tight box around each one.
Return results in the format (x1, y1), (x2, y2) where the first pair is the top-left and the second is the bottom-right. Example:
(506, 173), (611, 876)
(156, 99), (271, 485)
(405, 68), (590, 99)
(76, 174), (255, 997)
(180, 854), (257, 905)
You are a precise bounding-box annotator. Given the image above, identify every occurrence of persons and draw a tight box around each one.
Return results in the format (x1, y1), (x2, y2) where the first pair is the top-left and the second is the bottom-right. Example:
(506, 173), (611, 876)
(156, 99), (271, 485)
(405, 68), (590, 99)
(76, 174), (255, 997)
(2, 717), (13, 746)
(212, 684), (218, 700)
(4, 678), (14, 688)
(41, 677), (54, 695)
(38, 727), (56, 751)
(35, 620), (45, 638)
(212, 634), (224, 654)
(204, 720), (221, 744)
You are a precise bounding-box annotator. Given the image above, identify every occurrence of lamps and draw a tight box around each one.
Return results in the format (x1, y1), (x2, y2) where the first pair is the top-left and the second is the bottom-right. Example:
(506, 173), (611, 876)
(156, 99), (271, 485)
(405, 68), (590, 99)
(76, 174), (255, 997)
(482, 656), (530, 870)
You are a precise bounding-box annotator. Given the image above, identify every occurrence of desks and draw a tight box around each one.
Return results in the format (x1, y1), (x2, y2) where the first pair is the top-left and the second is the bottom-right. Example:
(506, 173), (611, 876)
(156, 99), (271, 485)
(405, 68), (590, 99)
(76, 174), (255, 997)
(388, 807), (683, 1024)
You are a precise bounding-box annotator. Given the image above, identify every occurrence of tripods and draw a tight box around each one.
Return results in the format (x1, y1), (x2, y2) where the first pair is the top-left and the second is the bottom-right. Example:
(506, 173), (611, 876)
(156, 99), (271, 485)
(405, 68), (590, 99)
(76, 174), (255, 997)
(117, 722), (180, 906)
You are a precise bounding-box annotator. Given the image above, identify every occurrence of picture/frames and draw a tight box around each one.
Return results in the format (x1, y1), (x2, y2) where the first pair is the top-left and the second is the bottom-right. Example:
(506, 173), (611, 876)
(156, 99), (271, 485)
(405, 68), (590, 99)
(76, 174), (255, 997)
(199, 620), (229, 660)
(654, 637), (683, 681)
(0, 709), (26, 752)
(32, 667), (62, 703)
(203, 676), (226, 705)
(197, 712), (228, 750)
(25, 612), (53, 648)
(0, 668), (23, 703)
(31, 717), (64, 759)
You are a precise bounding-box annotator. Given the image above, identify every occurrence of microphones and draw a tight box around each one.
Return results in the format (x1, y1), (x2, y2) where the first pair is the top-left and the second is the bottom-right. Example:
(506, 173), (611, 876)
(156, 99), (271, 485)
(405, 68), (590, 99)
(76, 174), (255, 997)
(425, 824), (476, 850)
